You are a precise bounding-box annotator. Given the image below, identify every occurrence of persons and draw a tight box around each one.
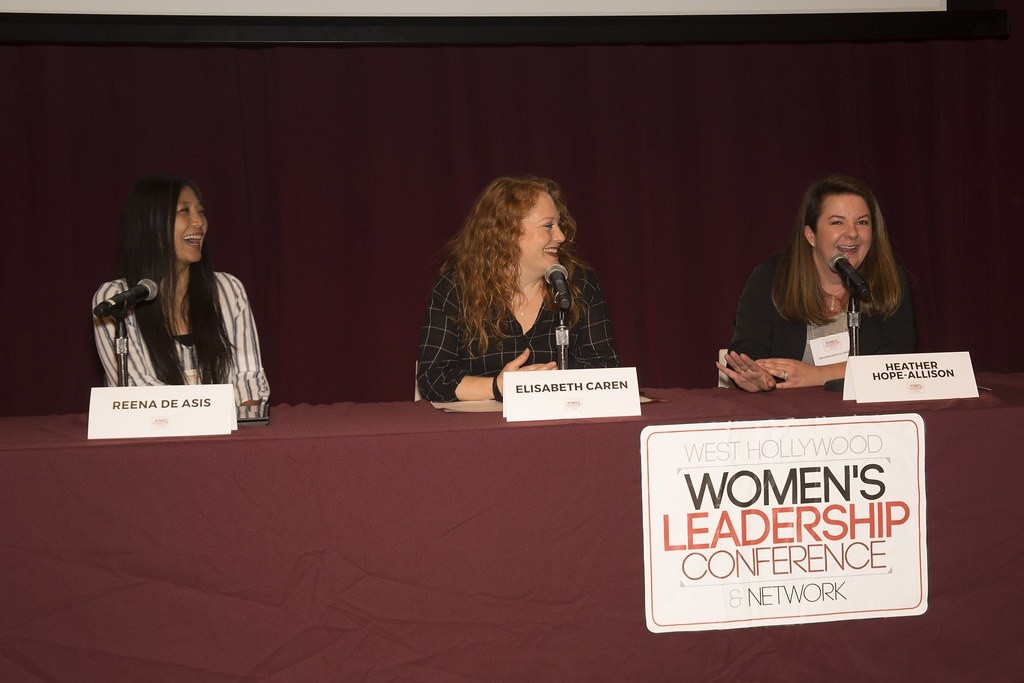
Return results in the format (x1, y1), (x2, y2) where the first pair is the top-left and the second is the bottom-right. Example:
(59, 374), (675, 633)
(713, 177), (918, 393)
(92, 172), (274, 422)
(415, 174), (623, 401)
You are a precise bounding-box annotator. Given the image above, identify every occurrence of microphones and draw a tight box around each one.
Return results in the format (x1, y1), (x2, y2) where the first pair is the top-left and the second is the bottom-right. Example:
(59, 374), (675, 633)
(544, 264), (572, 309)
(93, 278), (158, 318)
(829, 252), (872, 300)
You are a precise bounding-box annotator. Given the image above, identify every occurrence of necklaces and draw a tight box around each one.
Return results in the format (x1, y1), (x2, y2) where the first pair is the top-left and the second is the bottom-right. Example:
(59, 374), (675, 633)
(818, 284), (847, 311)
(508, 300), (532, 317)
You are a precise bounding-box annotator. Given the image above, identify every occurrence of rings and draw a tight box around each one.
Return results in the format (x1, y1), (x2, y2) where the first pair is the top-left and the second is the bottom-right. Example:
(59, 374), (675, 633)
(783, 370), (788, 383)
(744, 368), (751, 373)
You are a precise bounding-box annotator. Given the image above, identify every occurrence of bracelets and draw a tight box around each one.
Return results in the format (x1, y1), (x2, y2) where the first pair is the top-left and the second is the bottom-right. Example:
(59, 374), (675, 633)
(492, 377), (504, 403)
